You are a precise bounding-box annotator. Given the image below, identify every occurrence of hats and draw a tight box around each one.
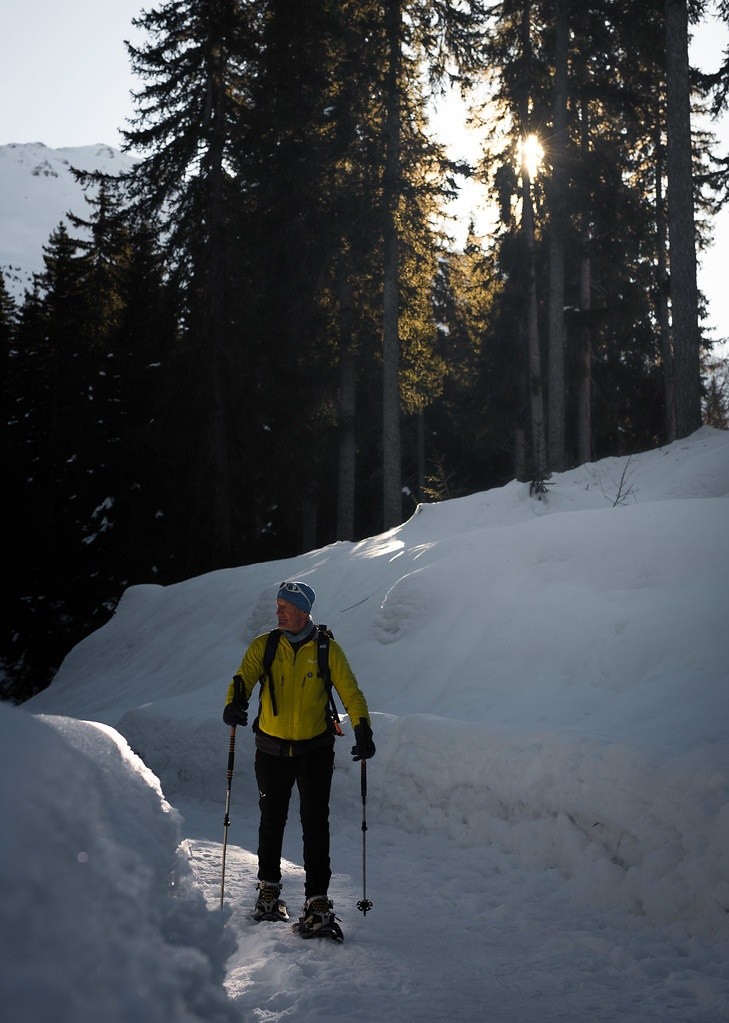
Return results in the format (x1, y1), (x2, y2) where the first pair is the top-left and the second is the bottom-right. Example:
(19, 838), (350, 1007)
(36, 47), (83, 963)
(277, 583), (315, 615)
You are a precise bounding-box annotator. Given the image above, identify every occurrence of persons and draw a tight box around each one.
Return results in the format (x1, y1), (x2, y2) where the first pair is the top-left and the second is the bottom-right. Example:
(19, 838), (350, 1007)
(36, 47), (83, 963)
(223, 582), (377, 944)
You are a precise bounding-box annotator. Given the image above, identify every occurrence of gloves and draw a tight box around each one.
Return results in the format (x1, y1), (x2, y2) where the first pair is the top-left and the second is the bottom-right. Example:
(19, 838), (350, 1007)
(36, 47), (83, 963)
(223, 702), (248, 727)
(350, 732), (376, 761)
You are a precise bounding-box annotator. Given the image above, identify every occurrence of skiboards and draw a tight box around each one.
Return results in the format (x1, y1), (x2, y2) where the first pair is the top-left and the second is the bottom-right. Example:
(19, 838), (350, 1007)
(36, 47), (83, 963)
(254, 898), (345, 944)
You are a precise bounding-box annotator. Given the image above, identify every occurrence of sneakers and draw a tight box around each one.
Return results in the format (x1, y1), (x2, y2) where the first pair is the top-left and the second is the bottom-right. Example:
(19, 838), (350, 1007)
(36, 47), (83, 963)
(306, 900), (330, 923)
(256, 884), (279, 913)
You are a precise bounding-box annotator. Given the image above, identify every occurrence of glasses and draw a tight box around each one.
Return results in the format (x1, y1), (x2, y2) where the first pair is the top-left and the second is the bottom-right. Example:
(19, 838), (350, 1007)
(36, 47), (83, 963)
(280, 582), (312, 608)
